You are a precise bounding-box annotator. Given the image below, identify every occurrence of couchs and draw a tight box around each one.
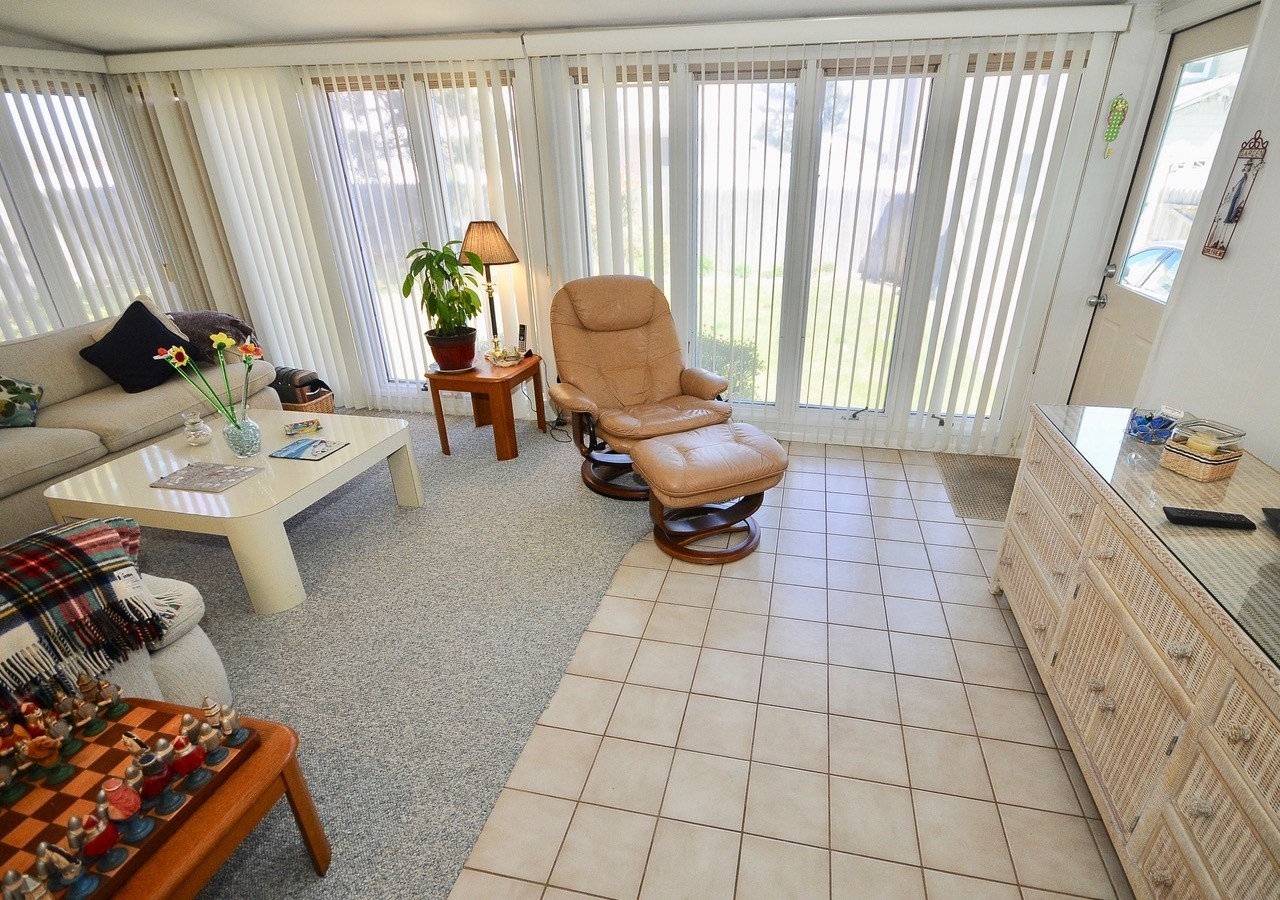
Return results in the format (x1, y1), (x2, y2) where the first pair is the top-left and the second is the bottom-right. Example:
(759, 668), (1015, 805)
(0, 518), (235, 718)
(0, 312), (282, 547)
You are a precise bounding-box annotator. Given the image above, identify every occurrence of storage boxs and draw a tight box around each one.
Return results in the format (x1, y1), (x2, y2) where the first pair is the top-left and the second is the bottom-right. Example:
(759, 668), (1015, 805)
(1161, 420), (1246, 483)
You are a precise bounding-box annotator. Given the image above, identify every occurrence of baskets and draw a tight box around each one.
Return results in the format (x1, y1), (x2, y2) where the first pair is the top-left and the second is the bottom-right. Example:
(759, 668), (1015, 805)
(1160, 434), (1243, 483)
(282, 391), (335, 414)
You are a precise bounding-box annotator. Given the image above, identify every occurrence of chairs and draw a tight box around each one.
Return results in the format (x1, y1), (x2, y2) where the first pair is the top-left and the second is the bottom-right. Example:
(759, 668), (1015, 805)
(550, 274), (789, 565)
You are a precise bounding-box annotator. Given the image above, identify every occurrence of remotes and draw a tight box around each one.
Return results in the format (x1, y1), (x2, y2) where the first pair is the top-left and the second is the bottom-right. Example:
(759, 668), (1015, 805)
(1163, 506), (1257, 530)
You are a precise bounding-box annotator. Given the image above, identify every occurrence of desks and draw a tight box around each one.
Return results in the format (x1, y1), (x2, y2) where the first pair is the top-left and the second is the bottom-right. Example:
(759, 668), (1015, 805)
(43, 407), (423, 616)
(0, 697), (329, 899)
(424, 354), (544, 461)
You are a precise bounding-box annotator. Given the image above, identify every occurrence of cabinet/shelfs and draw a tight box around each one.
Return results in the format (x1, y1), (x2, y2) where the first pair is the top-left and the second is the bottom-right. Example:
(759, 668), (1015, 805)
(991, 404), (1280, 899)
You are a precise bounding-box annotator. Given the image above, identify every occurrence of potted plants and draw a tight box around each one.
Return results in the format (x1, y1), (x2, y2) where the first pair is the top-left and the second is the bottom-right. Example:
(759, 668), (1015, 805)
(399, 239), (485, 374)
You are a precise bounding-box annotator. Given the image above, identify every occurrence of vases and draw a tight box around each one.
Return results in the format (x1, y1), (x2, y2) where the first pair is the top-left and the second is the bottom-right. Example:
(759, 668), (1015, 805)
(223, 407), (264, 459)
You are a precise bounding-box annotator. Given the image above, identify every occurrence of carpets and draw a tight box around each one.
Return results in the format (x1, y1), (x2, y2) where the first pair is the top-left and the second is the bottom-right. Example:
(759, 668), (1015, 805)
(933, 451), (1022, 522)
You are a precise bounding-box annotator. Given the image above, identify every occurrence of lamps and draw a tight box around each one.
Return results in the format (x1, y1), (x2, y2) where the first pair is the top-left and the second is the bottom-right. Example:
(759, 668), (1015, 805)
(457, 220), (519, 354)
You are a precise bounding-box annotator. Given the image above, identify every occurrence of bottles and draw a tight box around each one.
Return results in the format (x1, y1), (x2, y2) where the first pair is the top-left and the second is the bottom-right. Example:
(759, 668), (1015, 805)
(181, 411), (213, 447)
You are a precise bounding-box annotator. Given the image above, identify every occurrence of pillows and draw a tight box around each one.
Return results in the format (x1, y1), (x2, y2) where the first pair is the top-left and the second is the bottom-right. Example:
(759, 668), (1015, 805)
(0, 375), (45, 427)
(79, 294), (199, 396)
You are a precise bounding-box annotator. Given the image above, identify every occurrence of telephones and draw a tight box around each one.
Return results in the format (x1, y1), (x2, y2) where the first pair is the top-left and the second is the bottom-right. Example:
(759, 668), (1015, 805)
(517, 324), (527, 358)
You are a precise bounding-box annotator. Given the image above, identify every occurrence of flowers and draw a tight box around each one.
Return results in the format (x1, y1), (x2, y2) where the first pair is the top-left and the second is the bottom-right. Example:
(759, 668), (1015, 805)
(151, 333), (263, 427)
